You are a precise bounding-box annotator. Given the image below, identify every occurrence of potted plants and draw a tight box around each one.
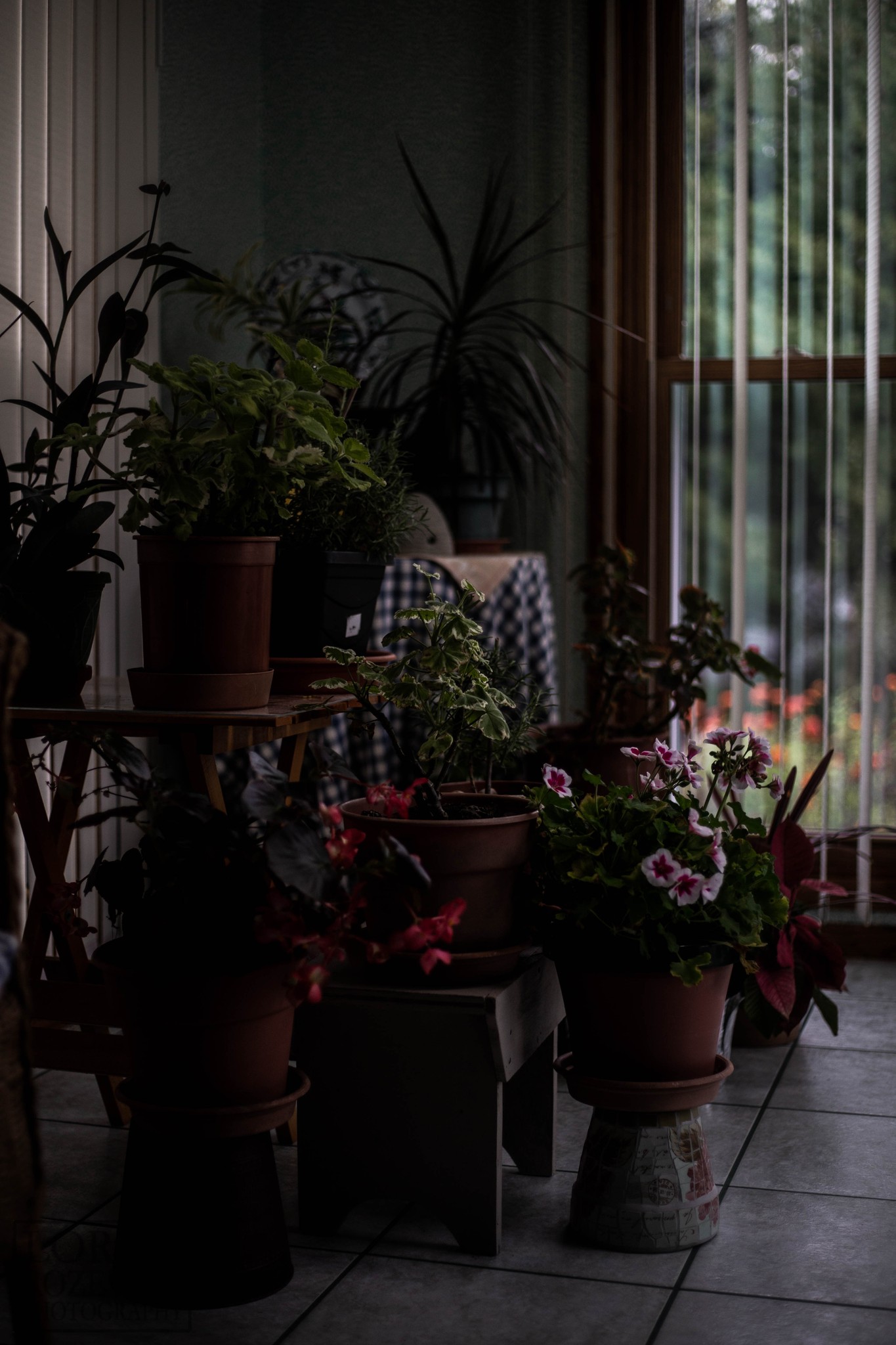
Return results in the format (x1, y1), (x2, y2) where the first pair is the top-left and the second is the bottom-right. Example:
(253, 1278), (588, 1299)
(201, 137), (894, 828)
(345, 133), (645, 545)
(0, 172), (216, 718)
(133, 319), (396, 706)
(732, 806), (895, 1044)
(557, 548), (764, 792)
(337, 594), (545, 964)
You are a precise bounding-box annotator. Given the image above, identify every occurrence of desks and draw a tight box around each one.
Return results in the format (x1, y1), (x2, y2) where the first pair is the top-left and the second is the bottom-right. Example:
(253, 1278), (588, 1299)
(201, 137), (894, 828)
(0, 689), (387, 1122)
(288, 548), (556, 806)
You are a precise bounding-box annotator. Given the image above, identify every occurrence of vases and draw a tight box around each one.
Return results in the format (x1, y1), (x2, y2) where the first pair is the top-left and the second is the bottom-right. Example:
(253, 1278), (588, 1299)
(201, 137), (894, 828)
(127, 944), (330, 1113)
(576, 953), (733, 1083)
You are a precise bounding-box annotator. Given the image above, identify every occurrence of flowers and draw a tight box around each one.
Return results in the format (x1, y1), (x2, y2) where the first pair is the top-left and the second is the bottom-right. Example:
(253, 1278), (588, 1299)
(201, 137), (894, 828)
(115, 755), (468, 1009)
(537, 730), (821, 956)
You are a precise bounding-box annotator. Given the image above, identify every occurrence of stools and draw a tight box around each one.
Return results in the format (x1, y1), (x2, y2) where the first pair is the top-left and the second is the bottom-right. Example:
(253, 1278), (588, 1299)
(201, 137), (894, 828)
(308, 965), (574, 1261)
